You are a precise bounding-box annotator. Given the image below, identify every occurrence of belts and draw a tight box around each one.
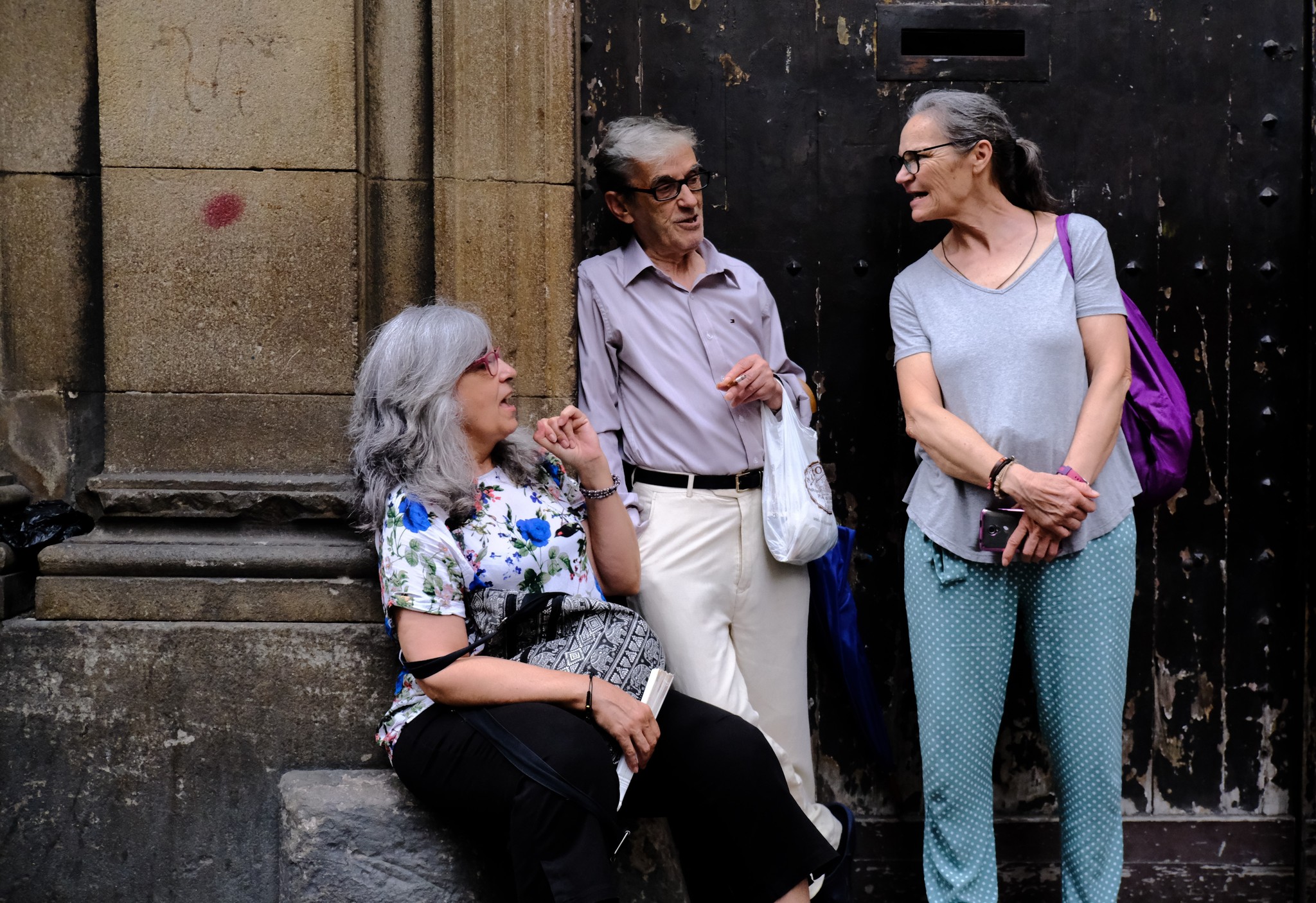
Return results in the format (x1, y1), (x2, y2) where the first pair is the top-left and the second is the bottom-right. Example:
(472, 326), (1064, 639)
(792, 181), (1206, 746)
(633, 467), (761, 490)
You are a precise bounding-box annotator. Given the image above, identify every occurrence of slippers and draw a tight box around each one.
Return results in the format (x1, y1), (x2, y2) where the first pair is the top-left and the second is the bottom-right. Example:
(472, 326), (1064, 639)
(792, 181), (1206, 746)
(810, 799), (854, 902)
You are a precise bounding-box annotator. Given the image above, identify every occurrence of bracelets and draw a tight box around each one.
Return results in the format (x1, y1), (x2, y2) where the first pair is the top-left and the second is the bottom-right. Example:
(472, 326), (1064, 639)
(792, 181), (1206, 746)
(585, 672), (595, 722)
(578, 475), (621, 501)
(986, 456), (1018, 501)
(1055, 465), (1090, 486)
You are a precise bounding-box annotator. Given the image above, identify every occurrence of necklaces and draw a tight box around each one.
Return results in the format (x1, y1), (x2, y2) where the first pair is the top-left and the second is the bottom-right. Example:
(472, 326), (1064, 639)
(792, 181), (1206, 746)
(941, 211), (1038, 291)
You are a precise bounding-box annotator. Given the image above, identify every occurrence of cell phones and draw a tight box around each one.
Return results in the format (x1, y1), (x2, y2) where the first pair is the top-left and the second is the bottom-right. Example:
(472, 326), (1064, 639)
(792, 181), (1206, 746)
(977, 508), (1064, 555)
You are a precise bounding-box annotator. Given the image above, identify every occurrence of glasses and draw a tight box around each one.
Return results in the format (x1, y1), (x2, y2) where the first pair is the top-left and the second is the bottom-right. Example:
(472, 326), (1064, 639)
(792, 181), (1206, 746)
(892, 135), (985, 174)
(632, 171), (713, 202)
(464, 344), (503, 377)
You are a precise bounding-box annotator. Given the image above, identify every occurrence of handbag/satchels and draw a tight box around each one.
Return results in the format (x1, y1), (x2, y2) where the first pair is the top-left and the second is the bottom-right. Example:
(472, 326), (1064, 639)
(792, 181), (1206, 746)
(760, 376), (836, 563)
(460, 585), (665, 801)
(1055, 212), (1193, 508)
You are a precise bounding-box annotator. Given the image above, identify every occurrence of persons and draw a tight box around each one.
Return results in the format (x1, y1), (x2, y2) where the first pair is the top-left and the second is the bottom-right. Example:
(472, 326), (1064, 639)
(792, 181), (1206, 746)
(575, 113), (840, 903)
(889, 90), (1145, 903)
(353, 302), (839, 903)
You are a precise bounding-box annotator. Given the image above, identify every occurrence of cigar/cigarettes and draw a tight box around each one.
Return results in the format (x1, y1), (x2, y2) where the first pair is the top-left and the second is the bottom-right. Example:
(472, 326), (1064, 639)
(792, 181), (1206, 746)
(727, 375), (746, 388)
